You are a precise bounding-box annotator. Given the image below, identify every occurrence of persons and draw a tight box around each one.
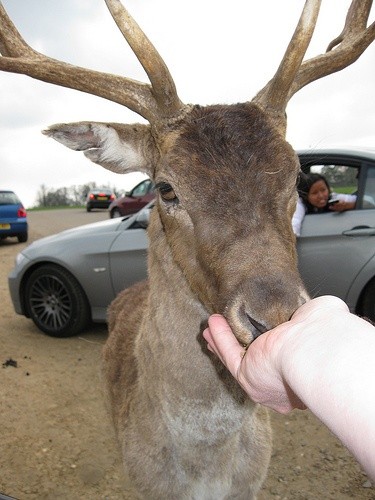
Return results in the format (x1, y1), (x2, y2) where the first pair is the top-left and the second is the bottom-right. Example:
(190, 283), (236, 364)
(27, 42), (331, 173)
(202, 294), (375, 481)
(291, 173), (358, 239)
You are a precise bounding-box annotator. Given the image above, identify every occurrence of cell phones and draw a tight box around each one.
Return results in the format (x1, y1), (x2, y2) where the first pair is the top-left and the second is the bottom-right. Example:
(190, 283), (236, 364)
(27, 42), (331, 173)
(329, 199), (339, 206)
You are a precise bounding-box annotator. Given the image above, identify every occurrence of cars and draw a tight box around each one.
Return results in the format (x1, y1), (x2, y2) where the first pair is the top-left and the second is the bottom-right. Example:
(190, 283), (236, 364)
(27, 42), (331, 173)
(6, 148), (375, 338)
(84, 187), (118, 212)
(108, 178), (156, 218)
(0, 190), (29, 246)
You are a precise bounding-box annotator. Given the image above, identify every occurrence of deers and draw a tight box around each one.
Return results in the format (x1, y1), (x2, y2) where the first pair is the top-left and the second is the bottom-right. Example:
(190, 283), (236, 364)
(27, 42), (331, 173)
(1, 0), (375, 500)
